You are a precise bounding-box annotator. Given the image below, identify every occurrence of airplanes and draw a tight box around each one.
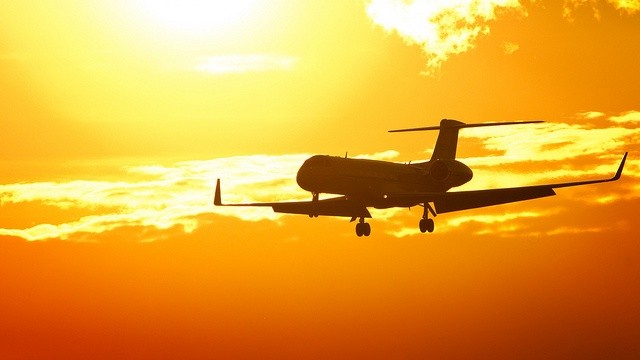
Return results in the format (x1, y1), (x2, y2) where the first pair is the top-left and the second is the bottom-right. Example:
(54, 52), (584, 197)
(205, 117), (629, 236)
